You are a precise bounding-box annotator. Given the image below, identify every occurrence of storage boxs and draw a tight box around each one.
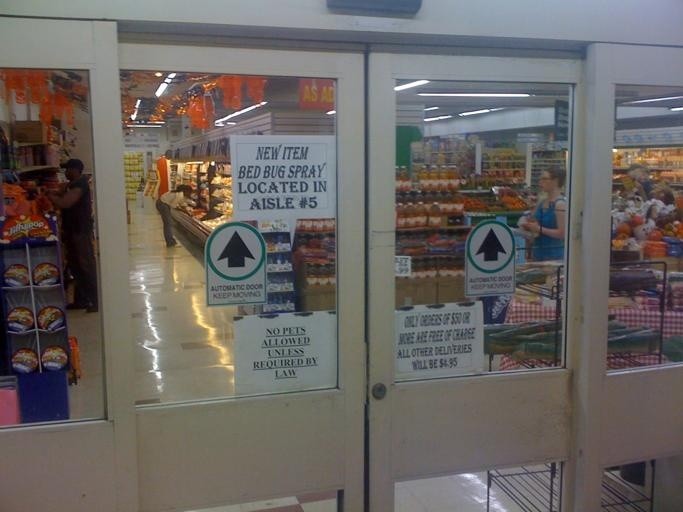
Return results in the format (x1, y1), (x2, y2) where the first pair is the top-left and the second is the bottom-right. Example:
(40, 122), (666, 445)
(12, 119), (47, 143)
(298, 274), (478, 312)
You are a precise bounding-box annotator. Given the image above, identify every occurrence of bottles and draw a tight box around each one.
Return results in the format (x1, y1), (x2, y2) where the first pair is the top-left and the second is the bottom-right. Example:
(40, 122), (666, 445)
(393, 163), (466, 229)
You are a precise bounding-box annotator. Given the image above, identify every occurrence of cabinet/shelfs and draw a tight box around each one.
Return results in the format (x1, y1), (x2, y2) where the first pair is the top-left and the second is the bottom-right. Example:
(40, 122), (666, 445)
(485, 202), (668, 512)
(0, 214), (72, 376)
(164, 112), (683, 263)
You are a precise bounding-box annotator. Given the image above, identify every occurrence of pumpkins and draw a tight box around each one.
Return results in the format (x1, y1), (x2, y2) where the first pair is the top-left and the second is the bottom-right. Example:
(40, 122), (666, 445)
(615, 215), (643, 240)
(648, 228), (662, 241)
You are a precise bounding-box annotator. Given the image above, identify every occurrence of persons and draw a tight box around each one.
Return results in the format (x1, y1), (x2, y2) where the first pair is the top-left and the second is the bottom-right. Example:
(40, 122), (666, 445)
(38, 157), (97, 314)
(516, 163), (565, 262)
(154, 181), (193, 247)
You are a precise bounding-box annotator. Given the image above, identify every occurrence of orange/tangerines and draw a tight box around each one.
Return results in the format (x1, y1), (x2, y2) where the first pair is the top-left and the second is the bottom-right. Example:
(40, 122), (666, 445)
(502, 195), (526, 208)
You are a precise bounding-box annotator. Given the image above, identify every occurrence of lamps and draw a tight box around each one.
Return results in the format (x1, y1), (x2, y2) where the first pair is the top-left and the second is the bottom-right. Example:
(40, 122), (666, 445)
(417, 86), (683, 105)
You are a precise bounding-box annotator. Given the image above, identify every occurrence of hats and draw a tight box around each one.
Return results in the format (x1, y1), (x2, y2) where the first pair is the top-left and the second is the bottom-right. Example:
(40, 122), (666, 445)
(58, 159), (85, 171)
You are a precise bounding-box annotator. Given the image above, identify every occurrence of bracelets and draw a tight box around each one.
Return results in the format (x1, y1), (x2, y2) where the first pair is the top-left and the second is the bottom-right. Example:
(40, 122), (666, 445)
(539, 224), (542, 234)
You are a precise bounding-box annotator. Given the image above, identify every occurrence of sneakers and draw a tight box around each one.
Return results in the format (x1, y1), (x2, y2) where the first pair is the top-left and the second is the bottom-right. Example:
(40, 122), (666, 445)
(66, 301), (98, 312)
